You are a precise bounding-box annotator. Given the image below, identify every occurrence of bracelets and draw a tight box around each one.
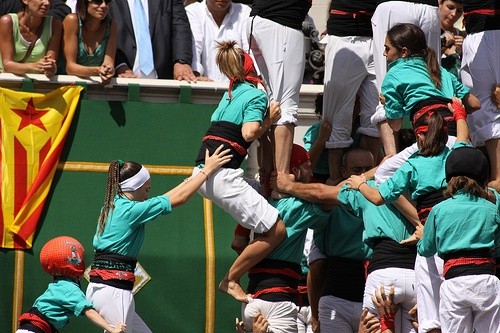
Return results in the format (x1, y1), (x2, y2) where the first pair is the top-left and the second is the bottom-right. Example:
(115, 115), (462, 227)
(357, 181), (367, 191)
(175, 59), (187, 64)
(199, 169), (210, 180)
(361, 173), (368, 183)
(379, 312), (396, 332)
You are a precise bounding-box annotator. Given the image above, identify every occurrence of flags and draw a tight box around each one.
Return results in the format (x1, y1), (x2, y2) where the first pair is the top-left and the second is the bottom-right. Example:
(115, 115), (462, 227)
(0, 81), (84, 253)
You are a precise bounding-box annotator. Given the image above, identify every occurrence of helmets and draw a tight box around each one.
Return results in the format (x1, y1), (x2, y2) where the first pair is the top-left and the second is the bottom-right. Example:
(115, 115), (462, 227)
(445, 147), (491, 186)
(290, 144), (311, 172)
(40, 236), (85, 277)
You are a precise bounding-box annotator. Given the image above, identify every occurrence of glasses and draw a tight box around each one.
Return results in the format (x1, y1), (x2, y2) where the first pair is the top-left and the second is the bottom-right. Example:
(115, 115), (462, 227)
(88, 0), (113, 5)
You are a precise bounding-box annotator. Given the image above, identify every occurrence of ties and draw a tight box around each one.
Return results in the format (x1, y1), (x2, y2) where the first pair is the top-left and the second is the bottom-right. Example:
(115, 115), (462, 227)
(132, 0), (155, 77)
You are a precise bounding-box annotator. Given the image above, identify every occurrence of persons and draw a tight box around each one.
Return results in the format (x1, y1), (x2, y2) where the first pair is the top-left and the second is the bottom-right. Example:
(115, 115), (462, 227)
(193, 39), (285, 303)
(59, 0), (122, 82)
(85, 143), (233, 333)
(1, 0), (63, 77)
(106, 0), (204, 84)
(235, 0), (500, 333)
(15, 236), (128, 333)
(187, 0), (255, 84)
